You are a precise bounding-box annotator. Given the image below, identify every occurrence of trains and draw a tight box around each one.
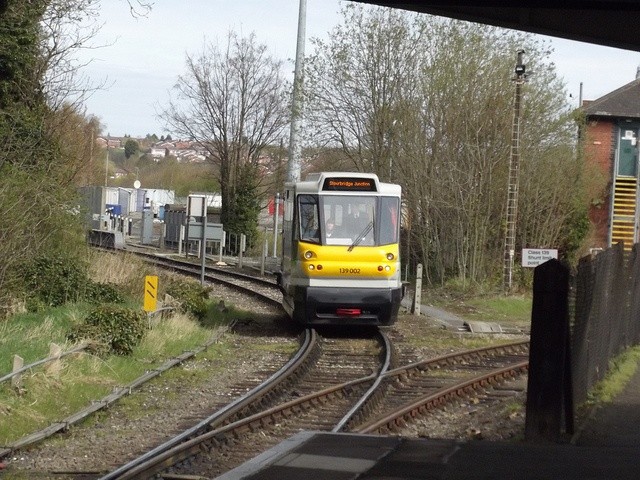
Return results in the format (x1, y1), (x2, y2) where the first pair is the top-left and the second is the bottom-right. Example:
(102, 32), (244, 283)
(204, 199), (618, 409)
(273, 171), (403, 327)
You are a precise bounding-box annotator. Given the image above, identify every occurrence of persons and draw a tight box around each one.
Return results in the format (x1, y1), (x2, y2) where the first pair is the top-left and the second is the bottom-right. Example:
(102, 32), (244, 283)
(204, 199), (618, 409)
(325, 218), (339, 238)
(153, 214), (165, 223)
